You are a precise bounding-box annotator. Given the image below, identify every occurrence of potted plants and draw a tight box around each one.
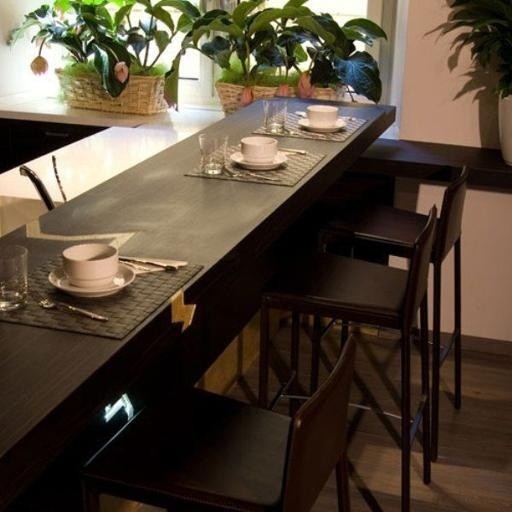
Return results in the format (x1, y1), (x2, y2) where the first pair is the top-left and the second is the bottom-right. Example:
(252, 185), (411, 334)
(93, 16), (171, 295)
(437, 0), (512, 167)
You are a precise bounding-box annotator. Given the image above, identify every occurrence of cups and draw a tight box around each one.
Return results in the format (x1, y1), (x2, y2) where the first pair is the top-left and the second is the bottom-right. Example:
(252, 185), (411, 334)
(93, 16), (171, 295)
(262, 97), (288, 134)
(198, 131), (226, 175)
(0, 244), (29, 313)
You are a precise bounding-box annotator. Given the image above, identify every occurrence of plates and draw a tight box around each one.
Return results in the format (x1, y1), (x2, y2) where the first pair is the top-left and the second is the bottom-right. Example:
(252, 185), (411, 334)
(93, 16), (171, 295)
(230, 151), (286, 171)
(298, 118), (345, 134)
(47, 263), (136, 301)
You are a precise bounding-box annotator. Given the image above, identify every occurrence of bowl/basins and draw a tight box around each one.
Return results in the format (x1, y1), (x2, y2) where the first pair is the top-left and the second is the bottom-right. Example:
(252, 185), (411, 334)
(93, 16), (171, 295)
(61, 243), (120, 288)
(306, 104), (340, 126)
(240, 135), (278, 163)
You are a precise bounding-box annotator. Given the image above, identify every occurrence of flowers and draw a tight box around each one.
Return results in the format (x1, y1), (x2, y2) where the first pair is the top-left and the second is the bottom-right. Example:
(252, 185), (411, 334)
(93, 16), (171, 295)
(162, 0), (390, 109)
(5, 1), (204, 99)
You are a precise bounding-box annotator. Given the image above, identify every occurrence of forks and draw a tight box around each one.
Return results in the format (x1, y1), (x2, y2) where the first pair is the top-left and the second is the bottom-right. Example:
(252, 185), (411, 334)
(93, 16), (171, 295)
(30, 287), (112, 323)
